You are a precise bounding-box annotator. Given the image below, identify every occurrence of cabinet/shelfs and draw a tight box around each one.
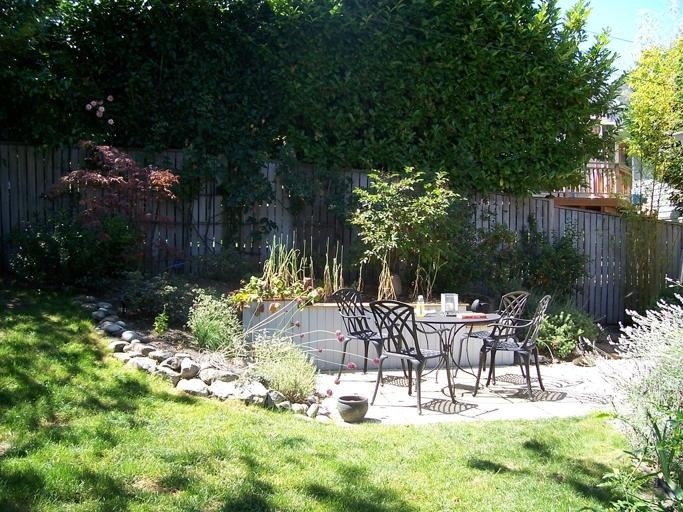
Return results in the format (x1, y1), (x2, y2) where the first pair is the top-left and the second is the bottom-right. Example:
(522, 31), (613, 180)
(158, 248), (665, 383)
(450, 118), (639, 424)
(237, 300), (514, 368)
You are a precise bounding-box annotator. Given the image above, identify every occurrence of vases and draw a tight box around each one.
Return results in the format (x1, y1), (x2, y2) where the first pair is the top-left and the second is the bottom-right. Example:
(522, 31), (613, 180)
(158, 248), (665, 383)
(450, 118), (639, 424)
(336, 393), (369, 422)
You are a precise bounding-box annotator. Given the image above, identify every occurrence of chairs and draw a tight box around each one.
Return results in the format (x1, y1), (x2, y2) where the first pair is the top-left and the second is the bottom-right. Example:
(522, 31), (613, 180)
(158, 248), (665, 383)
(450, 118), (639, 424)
(331, 288), (550, 413)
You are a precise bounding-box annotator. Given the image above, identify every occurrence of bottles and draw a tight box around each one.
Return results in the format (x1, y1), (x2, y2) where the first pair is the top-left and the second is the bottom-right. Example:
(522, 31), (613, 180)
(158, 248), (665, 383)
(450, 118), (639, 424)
(417, 295), (425, 319)
